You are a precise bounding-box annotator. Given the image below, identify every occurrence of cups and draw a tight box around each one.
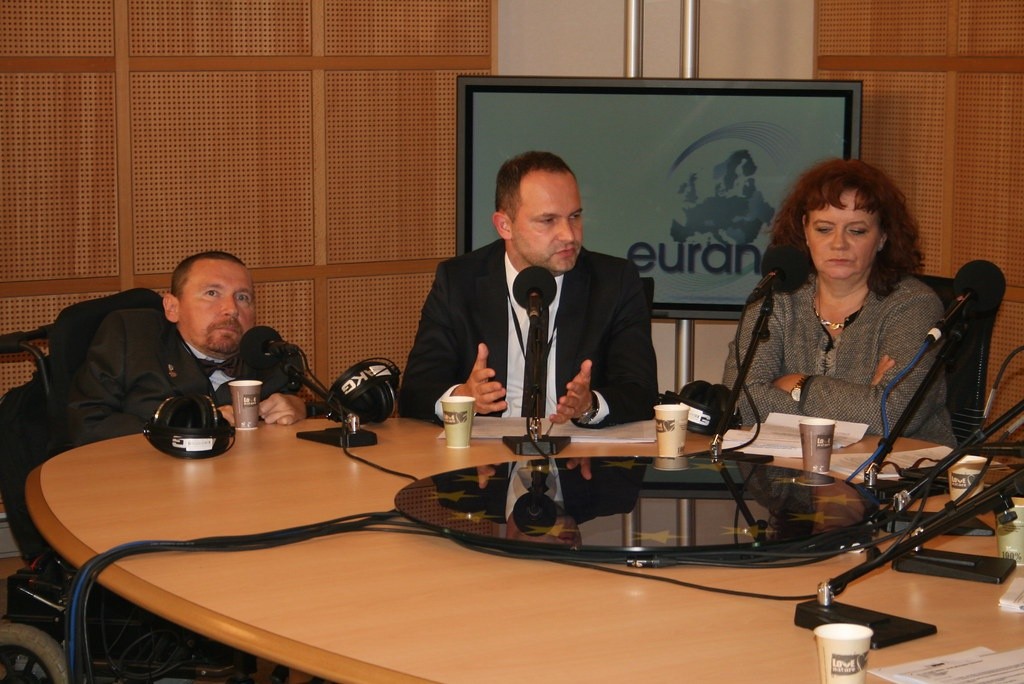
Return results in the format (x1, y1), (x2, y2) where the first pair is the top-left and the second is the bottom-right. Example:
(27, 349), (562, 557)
(814, 624), (873, 684)
(652, 458), (689, 472)
(996, 497), (1024, 567)
(798, 473), (835, 487)
(799, 419), (837, 472)
(228, 380), (263, 430)
(441, 396), (476, 449)
(949, 455), (987, 506)
(653, 403), (689, 457)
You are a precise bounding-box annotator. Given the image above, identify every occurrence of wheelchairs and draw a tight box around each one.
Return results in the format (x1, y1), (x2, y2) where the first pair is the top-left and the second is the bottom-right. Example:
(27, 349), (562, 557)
(0, 287), (292, 684)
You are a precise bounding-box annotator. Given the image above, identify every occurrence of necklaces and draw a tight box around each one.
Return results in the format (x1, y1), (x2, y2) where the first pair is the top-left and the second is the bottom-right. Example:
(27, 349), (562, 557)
(815, 306), (844, 329)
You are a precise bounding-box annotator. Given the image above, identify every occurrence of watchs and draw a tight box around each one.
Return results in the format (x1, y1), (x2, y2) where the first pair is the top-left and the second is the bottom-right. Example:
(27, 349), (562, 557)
(790, 375), (809, 402)
(578, 406), (595, 424)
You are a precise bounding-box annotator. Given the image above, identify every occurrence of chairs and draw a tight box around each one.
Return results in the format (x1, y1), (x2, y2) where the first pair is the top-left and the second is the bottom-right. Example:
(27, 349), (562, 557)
(901, 261), (1010, 455)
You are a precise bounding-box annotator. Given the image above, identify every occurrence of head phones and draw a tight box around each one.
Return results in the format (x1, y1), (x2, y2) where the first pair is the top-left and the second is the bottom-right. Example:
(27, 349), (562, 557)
(143, 393), (236, 460)
(659, 380), (742, 436)
(329, 356), (401, 423)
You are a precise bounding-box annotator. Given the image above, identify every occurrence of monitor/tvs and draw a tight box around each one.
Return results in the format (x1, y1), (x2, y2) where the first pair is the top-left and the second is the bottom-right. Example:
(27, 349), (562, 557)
(455, 74), (864, 321)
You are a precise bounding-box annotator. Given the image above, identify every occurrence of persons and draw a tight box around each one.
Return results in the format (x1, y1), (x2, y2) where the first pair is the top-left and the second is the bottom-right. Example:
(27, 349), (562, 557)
(397, 151), (661, 428)
(432, 456), (647, 544)
(66, 250), (308, 449)
(721, 159), (959, 452)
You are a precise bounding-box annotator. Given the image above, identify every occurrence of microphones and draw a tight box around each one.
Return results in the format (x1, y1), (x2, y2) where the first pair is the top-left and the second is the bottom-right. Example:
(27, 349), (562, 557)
(513, 265), (558, 326)
(746, 243), (810, 304)
(239, 326), (298, 369)
(513, 481), (557, 536)
(926, 260), (1006, 344)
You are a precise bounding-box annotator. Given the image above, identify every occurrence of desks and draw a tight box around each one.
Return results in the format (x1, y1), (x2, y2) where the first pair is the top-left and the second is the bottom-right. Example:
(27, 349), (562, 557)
(26, 412), (1024, 682)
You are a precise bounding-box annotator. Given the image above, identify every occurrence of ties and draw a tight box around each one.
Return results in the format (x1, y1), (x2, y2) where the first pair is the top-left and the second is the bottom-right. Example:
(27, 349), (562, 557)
(521, 307), (550, 418)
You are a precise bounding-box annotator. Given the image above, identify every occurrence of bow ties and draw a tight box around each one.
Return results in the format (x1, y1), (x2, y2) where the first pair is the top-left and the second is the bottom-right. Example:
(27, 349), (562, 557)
(195, 355), (240, 377)
